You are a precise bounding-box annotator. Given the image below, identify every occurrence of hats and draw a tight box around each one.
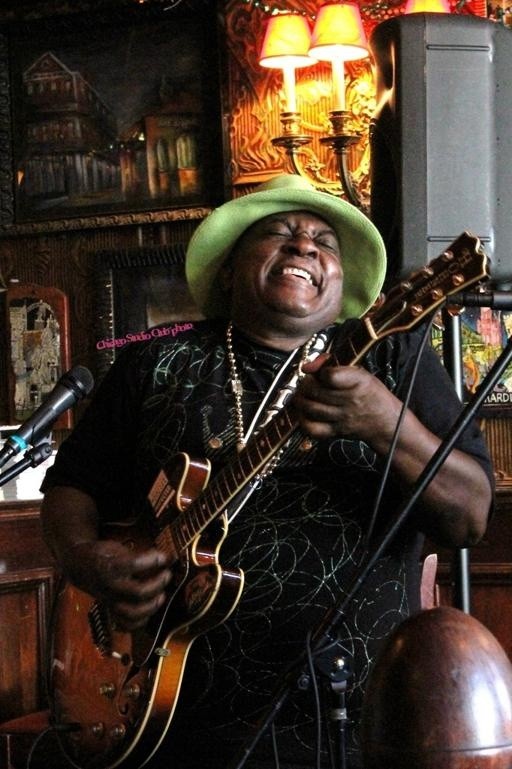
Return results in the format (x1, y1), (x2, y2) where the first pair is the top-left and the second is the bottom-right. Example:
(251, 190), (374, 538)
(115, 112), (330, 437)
(184, 173), (389, 321)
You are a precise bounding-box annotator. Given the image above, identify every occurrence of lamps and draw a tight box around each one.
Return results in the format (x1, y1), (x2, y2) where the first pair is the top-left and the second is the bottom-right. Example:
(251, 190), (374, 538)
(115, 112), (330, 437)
(255, 4), (374, 215)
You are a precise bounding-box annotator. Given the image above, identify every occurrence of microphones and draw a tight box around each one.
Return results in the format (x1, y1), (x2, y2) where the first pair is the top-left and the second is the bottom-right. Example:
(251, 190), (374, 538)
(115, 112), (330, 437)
(0, 365), (94, 469)
(446, 291), (512, 310)
